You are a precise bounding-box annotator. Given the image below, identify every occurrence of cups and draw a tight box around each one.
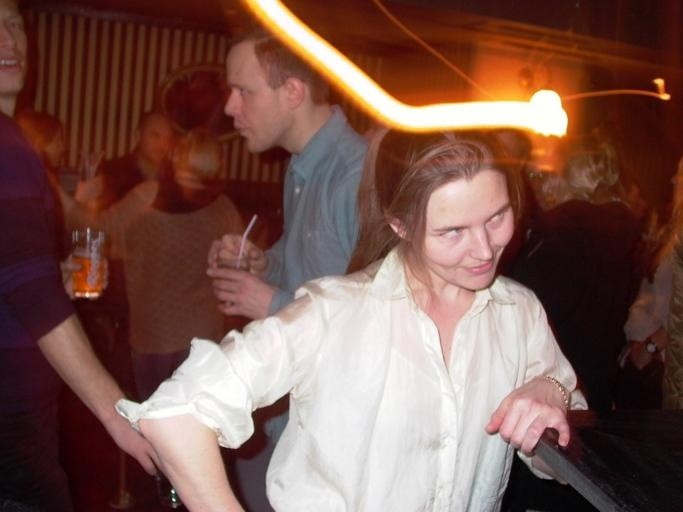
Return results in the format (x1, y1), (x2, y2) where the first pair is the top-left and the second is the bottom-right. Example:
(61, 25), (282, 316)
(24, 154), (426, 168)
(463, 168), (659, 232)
(70, 229), (106, 299)
(212, 246), (249, 319)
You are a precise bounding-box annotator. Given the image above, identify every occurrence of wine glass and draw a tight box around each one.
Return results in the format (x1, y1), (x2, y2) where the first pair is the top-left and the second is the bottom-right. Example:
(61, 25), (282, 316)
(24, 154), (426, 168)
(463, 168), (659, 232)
(81, 149), (103, 208)
